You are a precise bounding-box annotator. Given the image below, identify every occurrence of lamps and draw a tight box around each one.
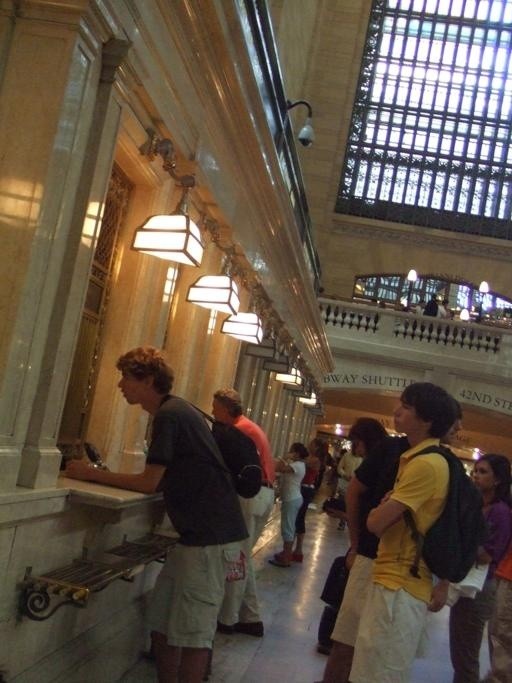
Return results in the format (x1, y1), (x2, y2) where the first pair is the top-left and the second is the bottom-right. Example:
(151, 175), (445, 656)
(459, 280), (490, 323)
(220, 297), (264, 346)
(185, 212), (241, 316)
(244, 326), (325, 416)
(129, 130), (206, 269)
(405, 268), (418, 309)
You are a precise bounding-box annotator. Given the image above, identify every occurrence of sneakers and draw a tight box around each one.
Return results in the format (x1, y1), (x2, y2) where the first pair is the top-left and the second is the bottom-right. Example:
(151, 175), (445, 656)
(268, 549), (304, 568)
(214, 620), (264, 637)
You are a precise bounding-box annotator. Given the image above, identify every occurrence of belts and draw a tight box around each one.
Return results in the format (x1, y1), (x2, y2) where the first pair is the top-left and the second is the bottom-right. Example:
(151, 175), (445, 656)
(300, 482), (315, 489)
(260, 480), (274, 488)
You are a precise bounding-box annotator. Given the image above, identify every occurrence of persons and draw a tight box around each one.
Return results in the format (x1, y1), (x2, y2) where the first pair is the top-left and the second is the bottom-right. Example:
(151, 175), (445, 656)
(270, 435), (333, 566)
(66, 343), (247, 680)
(208, 384), (275, 637)
(364, 292), (457, 342)
(314, 380), (511, 682)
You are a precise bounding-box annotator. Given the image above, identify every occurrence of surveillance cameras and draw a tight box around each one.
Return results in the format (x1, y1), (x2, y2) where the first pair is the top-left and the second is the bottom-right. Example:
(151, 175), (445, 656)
(297, 125), (316, 148)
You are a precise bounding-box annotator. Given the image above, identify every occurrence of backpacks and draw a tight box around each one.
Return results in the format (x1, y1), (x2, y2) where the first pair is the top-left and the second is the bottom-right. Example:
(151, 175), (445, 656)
(394, 444), (485, 583)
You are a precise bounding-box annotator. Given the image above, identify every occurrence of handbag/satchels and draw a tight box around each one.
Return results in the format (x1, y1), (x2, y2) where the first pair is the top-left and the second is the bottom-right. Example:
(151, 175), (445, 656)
(211, 420), (264, 500)
(319, 553), (350, 607)
